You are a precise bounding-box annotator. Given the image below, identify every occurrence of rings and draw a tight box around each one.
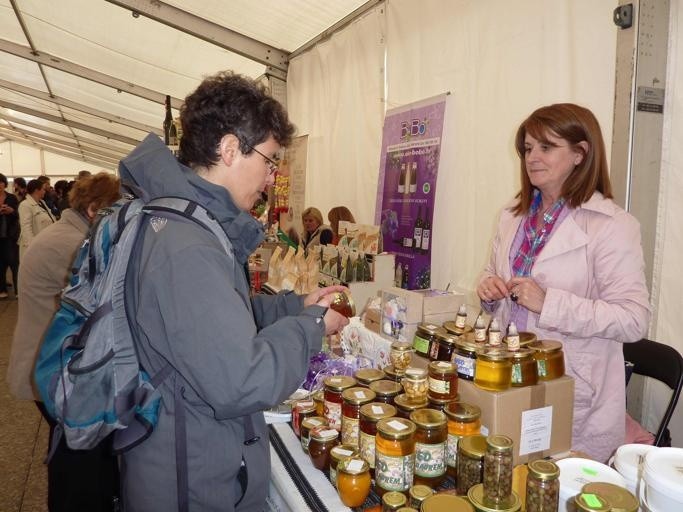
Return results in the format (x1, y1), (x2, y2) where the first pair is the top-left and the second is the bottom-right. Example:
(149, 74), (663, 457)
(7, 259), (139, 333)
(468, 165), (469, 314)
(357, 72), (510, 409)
(484, 289), (489, 294)
(510, 293), (519, 301)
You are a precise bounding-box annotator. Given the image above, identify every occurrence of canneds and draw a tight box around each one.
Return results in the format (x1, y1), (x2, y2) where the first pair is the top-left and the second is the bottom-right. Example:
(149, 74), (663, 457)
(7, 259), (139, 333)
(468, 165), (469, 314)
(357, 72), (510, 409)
(582, 482), (640, 512)
(483, 342), (507, 354)
(426, 333), (455, 363)
(473, 348), (514, 393)
(390, 342), (413, 369)
(409, 485), (433, 510)
(352, 369), (386, 386)
(427, 394), (460, 412)
(308, 427), (339, 471)
(442, 402), (481, 480)
(502, 331), (537, 348)
(468, 483), (522, 511)
(330, 444), (361, 488)
(336, 458), (371, 508)
(317, 289), (356, 318)
(508, 349), (539, 387)
(575, 491), (612, 512)
(456, 434), (486, 495)
(459, 332), (489, 345)
(525, 459), (560, 511)
(427, 360), (459, 400)
(301, 417), (330, 455)
(323, 375), (358, 442)
(443, 320), (473, 336)
(313, 391), (325, 417)
(409, 409), (448, 488)
(527, 340), (565, 381)
(383, 365), (405, 382)
(358, 402), (397, 480)
(380, 492), (407, 512)
(342, 386), (376, 453)
(291, 400), (317, 440)
(404, 369), (430, 404)
(375, 417), (416, 497)
(450, 342), (483, 382)
(413, 323), (448, 359)
(396, 506), (419, 512)
(369, 379), (403, 406)
(484, 434), (514, 504)
(394, 393), (429, 419)
(420, 494), (476, 512)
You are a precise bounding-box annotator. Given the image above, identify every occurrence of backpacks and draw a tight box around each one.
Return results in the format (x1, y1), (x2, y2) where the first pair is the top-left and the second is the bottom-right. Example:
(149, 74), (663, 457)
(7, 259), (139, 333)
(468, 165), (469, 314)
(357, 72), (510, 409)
(28, 193), (163, 463)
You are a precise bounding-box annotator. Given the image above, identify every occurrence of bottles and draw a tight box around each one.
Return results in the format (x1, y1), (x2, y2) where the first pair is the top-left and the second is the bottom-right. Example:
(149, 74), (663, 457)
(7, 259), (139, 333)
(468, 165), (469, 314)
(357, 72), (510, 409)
(406, 161), (420, 199)
(489, 317), (501, 345)
(476, 315), (486, 343)
(412, 204), (424, 249)
(168, 119), (178, 146)
(382, 435), (560, 512)
(396, 163), (407, 199)
(422, 206), (430, 253)
(508, 320), (521, 352)
(403, 264), (411, 287)
(396, 262), (404, 289)
(453, 303), (469, 328)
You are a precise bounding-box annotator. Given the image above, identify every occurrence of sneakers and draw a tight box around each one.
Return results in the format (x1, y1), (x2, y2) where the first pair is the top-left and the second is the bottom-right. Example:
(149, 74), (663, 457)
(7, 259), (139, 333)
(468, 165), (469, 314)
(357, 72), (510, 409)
(0, 292), (7, 298)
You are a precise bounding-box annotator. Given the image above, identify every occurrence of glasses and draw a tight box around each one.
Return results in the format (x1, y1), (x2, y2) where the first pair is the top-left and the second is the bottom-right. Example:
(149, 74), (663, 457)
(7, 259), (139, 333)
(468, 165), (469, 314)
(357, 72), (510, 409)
(232, 132), (282, 176)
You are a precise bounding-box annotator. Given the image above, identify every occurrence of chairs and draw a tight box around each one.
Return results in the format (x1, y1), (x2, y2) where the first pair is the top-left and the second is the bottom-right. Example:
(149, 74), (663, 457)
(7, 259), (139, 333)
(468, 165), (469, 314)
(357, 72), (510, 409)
(623, 338), (683, 446)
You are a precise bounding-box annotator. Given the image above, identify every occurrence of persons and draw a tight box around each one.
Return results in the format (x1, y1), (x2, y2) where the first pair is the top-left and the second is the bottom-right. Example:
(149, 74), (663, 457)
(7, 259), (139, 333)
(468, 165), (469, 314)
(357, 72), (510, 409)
(299, 207), (331, 258)
(5, 171), (124, 512)
(328, 207), (355, 245)
(475, 103), (652, 464)
(34, 70), (351, 512)
(1, 170), (92, 299)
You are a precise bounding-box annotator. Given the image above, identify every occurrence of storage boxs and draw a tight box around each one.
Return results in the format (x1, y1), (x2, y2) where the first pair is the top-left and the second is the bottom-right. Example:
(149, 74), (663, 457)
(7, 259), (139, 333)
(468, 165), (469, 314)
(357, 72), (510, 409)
(411, 351), (573, 466)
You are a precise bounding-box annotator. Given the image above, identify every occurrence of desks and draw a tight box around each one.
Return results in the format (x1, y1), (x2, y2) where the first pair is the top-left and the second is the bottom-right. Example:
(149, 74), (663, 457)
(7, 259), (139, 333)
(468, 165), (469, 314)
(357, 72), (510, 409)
(267, 424), (456, 512)
